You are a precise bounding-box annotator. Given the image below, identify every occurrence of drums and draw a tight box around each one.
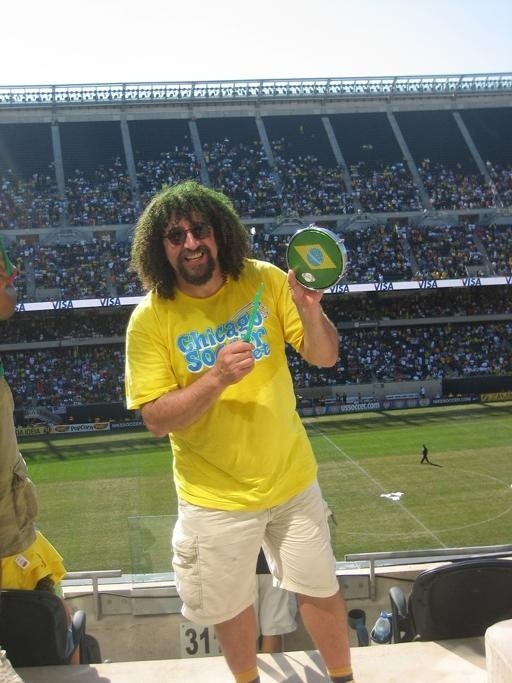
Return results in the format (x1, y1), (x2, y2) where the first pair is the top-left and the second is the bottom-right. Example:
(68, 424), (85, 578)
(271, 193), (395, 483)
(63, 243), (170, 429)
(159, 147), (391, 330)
(287, 224), (349, 292)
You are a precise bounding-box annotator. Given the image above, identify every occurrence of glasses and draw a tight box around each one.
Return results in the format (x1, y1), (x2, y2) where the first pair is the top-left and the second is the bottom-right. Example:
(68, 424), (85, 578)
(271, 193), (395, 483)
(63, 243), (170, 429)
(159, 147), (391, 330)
(160, 221), (214, 245)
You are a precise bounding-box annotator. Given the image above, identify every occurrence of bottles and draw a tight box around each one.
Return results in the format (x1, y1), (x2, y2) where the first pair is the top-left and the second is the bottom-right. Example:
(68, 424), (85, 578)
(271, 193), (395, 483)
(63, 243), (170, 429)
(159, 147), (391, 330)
(374, 611), (393, 643)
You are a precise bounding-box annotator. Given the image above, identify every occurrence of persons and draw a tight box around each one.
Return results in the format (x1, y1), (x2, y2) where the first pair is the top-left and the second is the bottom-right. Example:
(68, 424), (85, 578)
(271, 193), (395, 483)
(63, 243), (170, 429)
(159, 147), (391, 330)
(124, 177), (356, 683)
(0, 127), (511, 428)
(1, 227), (39, 560)
(252, 546), (299, 654)
(0, 530), (74, 632)
(420, 443), (429, 462)
